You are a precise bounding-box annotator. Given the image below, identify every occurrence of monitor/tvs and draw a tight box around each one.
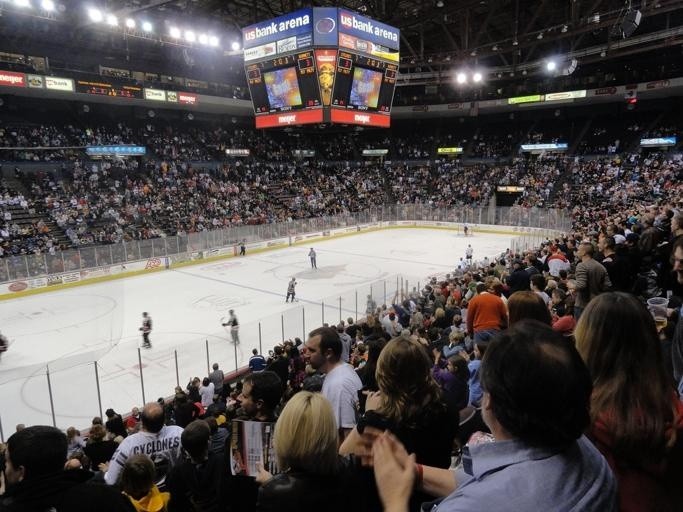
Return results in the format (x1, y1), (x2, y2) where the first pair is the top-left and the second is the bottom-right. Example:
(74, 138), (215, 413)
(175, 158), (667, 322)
(330, 49), (399, 115)
(246, 50), (323, 115)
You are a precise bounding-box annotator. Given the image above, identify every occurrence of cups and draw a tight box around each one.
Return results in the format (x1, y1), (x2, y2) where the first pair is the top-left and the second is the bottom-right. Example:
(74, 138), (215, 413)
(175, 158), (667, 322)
(646, 296), (669, 329)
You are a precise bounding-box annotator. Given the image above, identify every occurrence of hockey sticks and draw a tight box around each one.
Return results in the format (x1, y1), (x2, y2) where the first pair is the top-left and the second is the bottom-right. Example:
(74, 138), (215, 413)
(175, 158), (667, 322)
(294, 295), (299, 302)
(219, 316), (231, 338)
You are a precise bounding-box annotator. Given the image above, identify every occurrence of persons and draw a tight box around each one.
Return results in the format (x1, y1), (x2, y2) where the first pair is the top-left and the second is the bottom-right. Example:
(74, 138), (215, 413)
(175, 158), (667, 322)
(223, 308), (240, 344)
(158, 337), (326, 439)
(167, 421), (227, 512)
(124, 454), (170, 512)
(257, 390), (374, 511)
(64, 406), (142, 474)
(500, 123), (574, 235)
(105, 402), (186, 490)
(336, 295), (386, 386)
(338, 335), (460, 468)
(0, 117), (254, 274)
(574, 119), (683, 206)
(357, 322), (616, 512)
(305, 321), (363, 443)
(1, 423), (135, 512)
(575, 207), (683, 353)
(508, 234), (576, 338)
(308, 247), (318, 269)
(285, 276), (298, 304)
(139, 312), (152, 349)
(387, 236), (508, 402)
(254, 126), (500, 240)
(575, 291), (683, 512)
